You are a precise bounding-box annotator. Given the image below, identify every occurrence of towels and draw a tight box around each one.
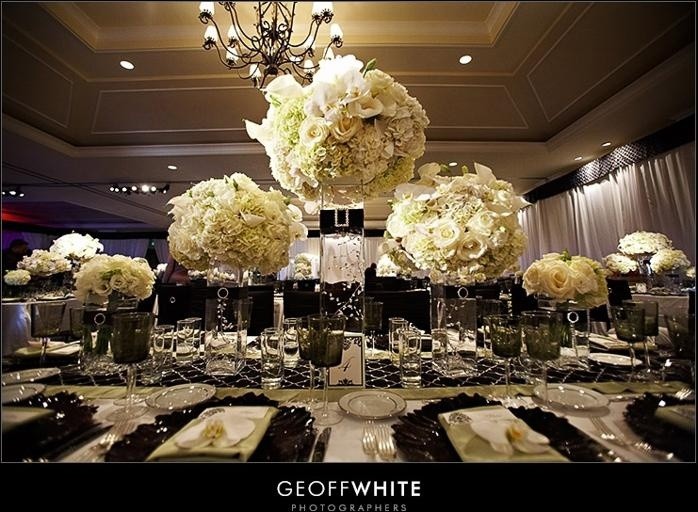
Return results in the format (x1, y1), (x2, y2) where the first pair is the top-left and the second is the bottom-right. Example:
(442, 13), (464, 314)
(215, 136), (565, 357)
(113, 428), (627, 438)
(654, 403), (695, 431)
(146, 405), (278, 462)
(438, 404), (570, 463)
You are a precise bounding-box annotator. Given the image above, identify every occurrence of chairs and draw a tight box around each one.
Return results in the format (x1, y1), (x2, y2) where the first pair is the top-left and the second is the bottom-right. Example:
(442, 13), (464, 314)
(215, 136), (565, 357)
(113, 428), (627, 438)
(137, 290), (156, 311)
(83, 311), (135, 332)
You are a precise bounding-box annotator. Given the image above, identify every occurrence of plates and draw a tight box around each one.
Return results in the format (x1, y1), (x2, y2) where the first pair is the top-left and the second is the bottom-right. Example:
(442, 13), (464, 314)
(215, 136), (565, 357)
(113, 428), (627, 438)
(338, 388), (407, 418)
(535, 382), (609, 411)
(1, 367), (60, 405)
(588, 351), (641, 370)
(144, 382), (216, 411)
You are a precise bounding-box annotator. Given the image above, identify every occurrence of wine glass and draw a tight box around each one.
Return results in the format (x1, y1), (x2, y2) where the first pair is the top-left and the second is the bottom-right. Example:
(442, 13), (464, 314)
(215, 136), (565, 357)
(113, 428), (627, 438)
(610, 300), (659, 383)
(70, 306), (86, 365)
(479, 299), (521, 398)
(111, 314), (151, 421)
(361, 301), (383, 353)
(522, 310), (562, 385)
(31, 302), (65, 367)
(297, 313), (343, 426)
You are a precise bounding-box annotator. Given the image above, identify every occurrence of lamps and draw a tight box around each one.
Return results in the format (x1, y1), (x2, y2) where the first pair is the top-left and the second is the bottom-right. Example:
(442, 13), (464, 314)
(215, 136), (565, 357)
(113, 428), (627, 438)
(108, 184), (170, 195)
(194, 0), (344, 95)
(2, 185), (25, 199)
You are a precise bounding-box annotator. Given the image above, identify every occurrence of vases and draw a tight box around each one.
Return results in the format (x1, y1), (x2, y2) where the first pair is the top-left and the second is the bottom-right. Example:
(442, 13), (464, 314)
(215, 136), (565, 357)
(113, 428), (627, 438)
(79, 289), (136, 381)
(537, 289), (593, 357)
(204, 254), (255, 376)
(429, 274), (479, 379)
(318, 177), (368, 388)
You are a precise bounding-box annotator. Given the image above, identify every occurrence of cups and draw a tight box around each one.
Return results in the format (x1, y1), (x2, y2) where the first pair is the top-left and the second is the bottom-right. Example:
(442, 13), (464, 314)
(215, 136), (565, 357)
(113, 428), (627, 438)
(260, 318), (298, 388)
(386, 316), (423, 388)
(140, 316), (201, 385)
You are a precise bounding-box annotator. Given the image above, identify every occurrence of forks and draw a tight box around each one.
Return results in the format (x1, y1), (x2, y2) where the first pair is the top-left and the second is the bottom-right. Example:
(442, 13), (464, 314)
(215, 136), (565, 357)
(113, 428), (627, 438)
(83, 415), (131, 462)
(375, 423), (397, 463)
(590, 415), (650, 464)
(361, 420), (376, 458)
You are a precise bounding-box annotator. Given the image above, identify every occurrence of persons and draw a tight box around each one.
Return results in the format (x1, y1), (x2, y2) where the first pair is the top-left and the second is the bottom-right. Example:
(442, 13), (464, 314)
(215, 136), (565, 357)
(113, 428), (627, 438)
(2, 239), (30, 270)
(162, 253), (191, 284)
(364, 263), (376, 278)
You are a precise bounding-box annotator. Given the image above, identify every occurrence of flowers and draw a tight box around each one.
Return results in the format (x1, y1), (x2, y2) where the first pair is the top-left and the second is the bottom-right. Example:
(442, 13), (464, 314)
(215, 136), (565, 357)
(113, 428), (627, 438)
(4, 231), (164, 306)
(165, 172), (312, 280)
(243, 56), (430, 196)
(522, 231), (696, 309)
(377, 162), (530, 287)
